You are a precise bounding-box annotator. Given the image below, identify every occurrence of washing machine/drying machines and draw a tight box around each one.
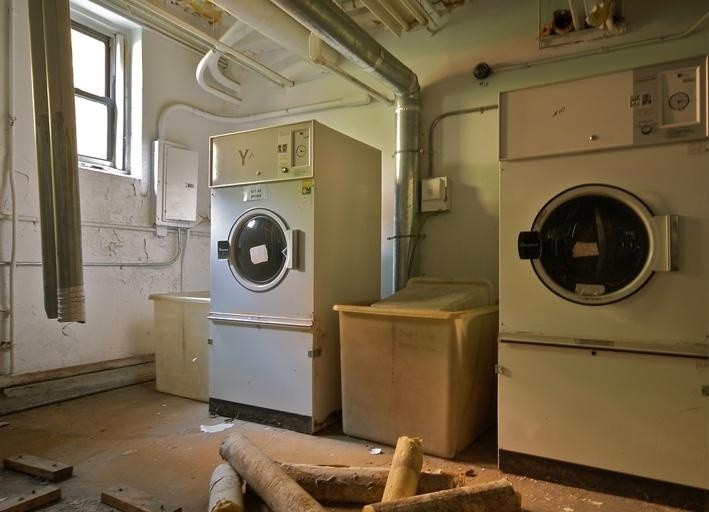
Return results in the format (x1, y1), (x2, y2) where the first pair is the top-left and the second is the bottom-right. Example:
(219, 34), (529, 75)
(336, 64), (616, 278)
(209, 120), (381, 435)
(498, 54), (709, 511)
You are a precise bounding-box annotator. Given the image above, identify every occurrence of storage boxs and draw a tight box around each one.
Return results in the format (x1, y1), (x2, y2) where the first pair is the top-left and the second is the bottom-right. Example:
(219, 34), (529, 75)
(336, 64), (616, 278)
(330, 298), (500, 459)
(367, 276), (496, 313)
(146, 289), (211, 402)
